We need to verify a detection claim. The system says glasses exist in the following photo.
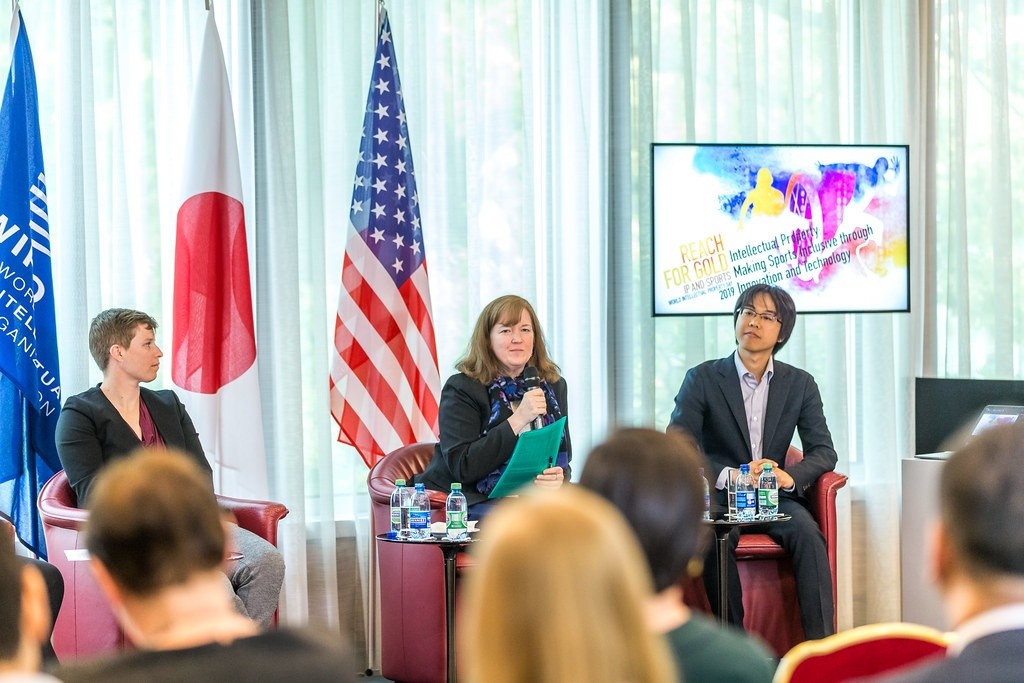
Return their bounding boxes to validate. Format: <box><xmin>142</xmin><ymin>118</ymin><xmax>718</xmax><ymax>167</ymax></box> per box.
<box><xmin>738</xmin><ymin>307</ymin><xmax>782</xmax><ymax>325</ymax></box>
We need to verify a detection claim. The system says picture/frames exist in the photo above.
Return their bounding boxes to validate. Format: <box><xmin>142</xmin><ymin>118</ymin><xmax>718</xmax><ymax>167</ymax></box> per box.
<box><xmin>649</xmin><ymin>141</ymin><xmax>912</xmax><ymax>319</ymax></box>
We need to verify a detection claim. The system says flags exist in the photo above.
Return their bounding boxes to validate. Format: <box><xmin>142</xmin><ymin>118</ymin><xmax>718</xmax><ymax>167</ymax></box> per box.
<box><xmin>161</xmin><ymin>0</ymin><xmax>272</xmax><ymax>504</ymax></box>
<box><xmin>0</xmin><ymin>1</ymin><xmax>64</xmax><ymax>569</ymax></box>
<box><xmin>328</xmin><ymin>1</ymin><xmax>444</xmax><ymax>470</ymax></box>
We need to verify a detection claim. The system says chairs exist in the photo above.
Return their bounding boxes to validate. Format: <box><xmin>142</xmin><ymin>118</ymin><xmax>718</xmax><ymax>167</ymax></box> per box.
<box><xmin>770</xmin><ymin>621</ymin><xmax>951</xmax><ymax>683</ymax></box>
<box><xmin>366</xmin><ymin>442</ymin><xmax>476</xmax><ymax>683</ymax></box>
<box><xmin>36</xmin><ymin>468</ymin><xmax>290</xmax><ymax>670</ymax></box>
<box><xmin>666</xmin><ymin>425</ymin><xmax>848</xmax><ymax>660</ymax></box>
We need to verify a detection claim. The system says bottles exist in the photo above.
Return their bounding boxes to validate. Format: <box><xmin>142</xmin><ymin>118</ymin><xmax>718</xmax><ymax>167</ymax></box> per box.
<box><xmin>699</xmin><ymin>467</ymin><xmax>711</xmax><ymax>520</ymax></box>
<box><xmin>408</xmin><ymin>483</ymin><xmax>430</xmax><ymax>539</ymax></box>
<box><xmin>445</xmin><ymin>483</ymin><xmax>467</xmax><ymax>539</ymax></box>
<box><xmin>757</xmin><ymin>462</ymin><xmax>779</xmax><ymax>515</ymax></box>
<box><xmin>389</xmin><ymin>479</ymin><xmax>411</xmax><ymax>537</ymax></box>
<box><xmin>736</xmin><ymin>465</ymin><xmax>756</xmax><ymax>518</ymax></box>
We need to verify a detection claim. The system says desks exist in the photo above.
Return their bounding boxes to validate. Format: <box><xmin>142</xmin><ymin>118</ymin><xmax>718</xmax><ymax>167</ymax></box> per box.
<box><xmin>696</xmin><ymin>510</ymin><xmax>791</xmax><ymax>634</ymax></box>
<box><xmin>375</xmin><ymin>531</ymin><xmax>479</xmax><ymax>682</ymax></box>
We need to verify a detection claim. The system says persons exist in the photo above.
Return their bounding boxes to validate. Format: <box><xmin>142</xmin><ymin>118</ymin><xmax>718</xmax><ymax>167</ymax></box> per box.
<box><xmin>451</xmin><ymin>426</ymin><xmax>773</xmax><ymax>683</ymax></box>
<box><xmin>0</xmin><ymin>450</ymin><xmax>364</xmax><ymax>683</ymax></box>
<box><xmin>54</xmin><ymin>307</ymin><xmax>284</xmax><ymax>634</ymax></box>
<box><xmin>663</xmin><ymin>283</ymin><xmax>840</xmax><ymax>653</ymax></box>
<box><xmin>879</xmin><ymin>424</ymin><xmax>1024</xmax><ymax>683</ymax></box>
<box><xmin>413</xmin><ymin>296</ymin><xmax>571</xmax><ymax>528</ymax></box>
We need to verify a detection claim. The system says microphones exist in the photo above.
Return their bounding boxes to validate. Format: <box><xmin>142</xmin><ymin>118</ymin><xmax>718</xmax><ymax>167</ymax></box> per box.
<box><xmin>524</xmin><ymin>366</ymin><xmax>543</xmax><ymax>429</ymax></box>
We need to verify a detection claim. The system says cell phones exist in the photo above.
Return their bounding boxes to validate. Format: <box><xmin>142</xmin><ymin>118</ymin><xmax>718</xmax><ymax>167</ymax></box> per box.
<box><xmin>223</xmin><ymin>551</ymin><xmax>244</xmax><ymax>561</ymax></box>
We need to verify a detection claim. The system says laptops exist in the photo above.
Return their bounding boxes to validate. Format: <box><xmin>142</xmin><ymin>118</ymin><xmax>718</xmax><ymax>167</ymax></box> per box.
<box><xmin>915</xmin><ymin>404</ymin><xmax>1024</xmax><ymax>461</ymax></box>
